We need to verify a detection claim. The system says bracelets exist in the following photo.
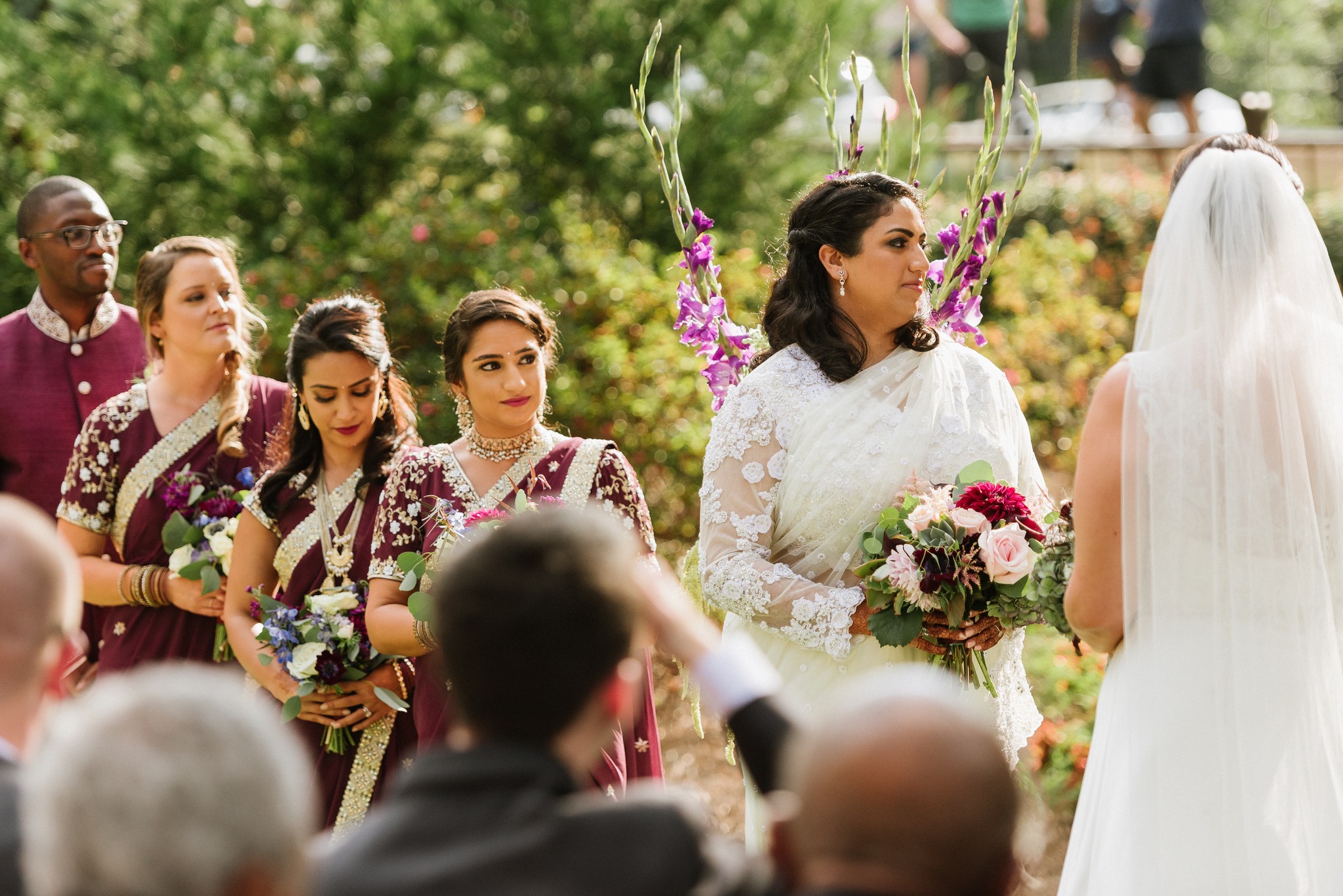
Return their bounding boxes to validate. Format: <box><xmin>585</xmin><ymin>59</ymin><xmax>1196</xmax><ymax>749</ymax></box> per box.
<box><xmin>412</xmin><ymin>618</ymin><xmax>441</xmax><ymax>652</ymax></box>
<box><xmin>387</xmin><ymin>656</ymin><xmax>417</xmax><ymax>699</ymax></box>
<box><xmin>118</xmin><ymin>564</ymin><xmax>169</xmax><ymax>608</ymax></box>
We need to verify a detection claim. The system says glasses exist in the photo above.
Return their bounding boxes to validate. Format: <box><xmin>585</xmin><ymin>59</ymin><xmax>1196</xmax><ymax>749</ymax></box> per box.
<box><xmin>27</xmin><ymin>220</ymin><xmax>127</xmax><ymax>250</ymax></box>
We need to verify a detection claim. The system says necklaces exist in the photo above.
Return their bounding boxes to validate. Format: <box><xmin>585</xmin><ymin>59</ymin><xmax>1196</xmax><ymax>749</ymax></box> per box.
<box><xmin>470</xmin><ymin>423</ymin><xmax>541</xmax><ymax>451</ymax></box>
<box><xmin>315</xmin><ymin>464</ymin><xmax>370</xmax><ymax>595</ymax></box>
<box><xmin>466</xmin><ymin>435</ymin><xmax>542</xmax><ymax>461</ymax></box>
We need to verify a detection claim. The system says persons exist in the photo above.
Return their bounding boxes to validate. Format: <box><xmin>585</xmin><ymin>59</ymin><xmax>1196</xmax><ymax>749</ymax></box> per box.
<box><xmin>769</xmin><ymin>687</ymin><xmax>1023</xmax><ymax>895</ymax></box>
<box><xmin>18</xmin><ymin>662</ymin><xmax>323</xmax><ymax>896</ymax></box>
<box><xmin>1055</xmin><ymin>135</ymin><xmax>1343</xmax><ymax>895</ymax></box>
<box><xmin>220</xmin><ymin>293</ymin><xmax>424</xmax><ymax>838</ymax></box>
<box><xmin>696</xmin><ymin>175</ymin><xmax>1060</xmax><ymax>896</ymax></box>
<box><xmin>0</xmin><ymin>490</ymin><xmax>89</xmax><ymax>896</ymax></box>
<box><xmin>364</xmin><ymin>288</ymin><xmax>666</xmax><ymax>799</ymax></box>
<box><xmin>55</xmin><ymin>232</ymin><xmax>293</xmax><ymax>669</ymax></box>
<box><xmin>310</xmin><ymin>500</ymin><xmax>803</xmax><ymax>896</ymax></box>
<box><xmin>1088</xmin><ymin>0</ymin><xmax>1207</xmax><ymax>139</ymax></box>
<box><xmin>0</xmin><ymin>173</ymin><xmax>160</xmax><ymax>662</ymax></box>
<box><xmin>886</xmin><ymin>0</ymin><xmax>1050</xmax><ymax>137</ymax></box>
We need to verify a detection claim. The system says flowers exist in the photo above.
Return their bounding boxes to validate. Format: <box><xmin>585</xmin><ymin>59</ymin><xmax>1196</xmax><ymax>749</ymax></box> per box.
<box><xmin>159</xmin><ymin>459</ymin><xmax>266</xmax><ymax>671</ymax></box>
<box><xmin>243</xmin><ymin>566</ymin><xmax>413</xmax><ymax>760</ymax></box>
<box><xmin>625</xmin><ymin>0</ymin><xmax>1046</xmax><ymax>408</ymax></box>
<box><xmin>860</xmin><ymin>462</ymin><xmax>1047</xmax><ymax>711</ymax></box>
<box><xmin>399</xmin><ymin>460</ymin><xmax>589</xmax><ymax>630</ymax></box>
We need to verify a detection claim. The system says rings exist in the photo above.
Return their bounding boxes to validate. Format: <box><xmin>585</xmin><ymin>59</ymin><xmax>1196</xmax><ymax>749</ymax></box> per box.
<box><xmin>363</xmin><ymin>706</ymin><xmax>372</xmax><ymax>717</ymax></box>
<box><xmin>920</xmin><ymin>627</ymin><xmax>930</xmax><ymax>638</ymax></box>
<box><xmin>993</xmin><ymin>624</ymin><xmax>1003</xmax><ymax>636</ymax></box>
<box><xmin>209</xmin><ymin>599</ymin><xmax>214</xmax><ymax>608</ymax></box>
